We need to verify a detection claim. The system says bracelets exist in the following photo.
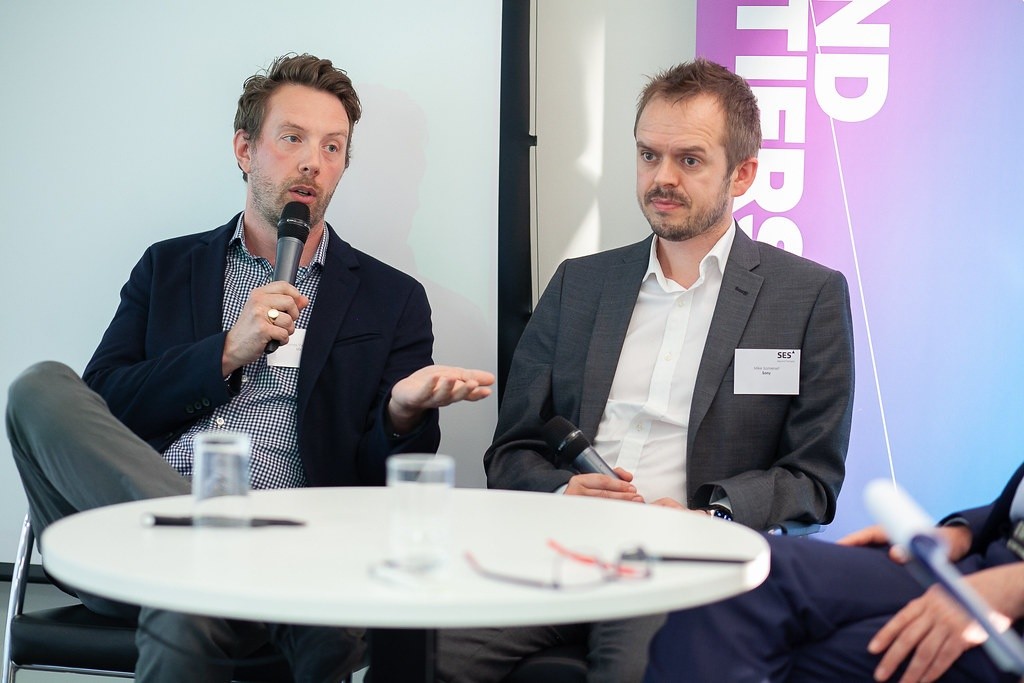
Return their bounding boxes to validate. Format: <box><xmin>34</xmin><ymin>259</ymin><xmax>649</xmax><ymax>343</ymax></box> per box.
<box><xmin>224</xmin><ymin>373</ymin><xmax>232</xmax><ymax>382</ymax></box>
<box><xmin>391</xmin><ymin>432</ymin><xmax>402</xmax><ymax>438</ymax></box>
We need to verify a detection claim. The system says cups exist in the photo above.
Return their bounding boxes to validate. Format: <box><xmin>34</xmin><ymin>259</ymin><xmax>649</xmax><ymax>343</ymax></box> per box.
<box><xmin>386</xmin><ymin>455</ymin><xmax>452</xmax><ymax>557</ymax></box>
<box><xmin>193</xmin><ymin>430</ymin><xmax>255</xmax><ymax>550</ymax></box>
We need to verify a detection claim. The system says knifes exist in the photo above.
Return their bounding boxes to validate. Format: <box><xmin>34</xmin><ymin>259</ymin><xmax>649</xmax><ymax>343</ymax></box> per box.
<box><xmin>146</xmin><ymin>513</ymin><xmax>302</xmax><ymax>528</ymax></box>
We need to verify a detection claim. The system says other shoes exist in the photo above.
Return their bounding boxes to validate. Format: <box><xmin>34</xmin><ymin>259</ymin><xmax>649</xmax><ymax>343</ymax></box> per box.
<box><xmin>276</xmin><ymin>627</ymin><xmax>368</xmax><ymax>683</ymax></box>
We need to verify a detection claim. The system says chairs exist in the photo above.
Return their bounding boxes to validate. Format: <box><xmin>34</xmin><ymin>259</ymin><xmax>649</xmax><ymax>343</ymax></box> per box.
<box><xmin>1</xmin><ymin>513</ymin><xmax>141</xmax><ymax>683</ymax></box>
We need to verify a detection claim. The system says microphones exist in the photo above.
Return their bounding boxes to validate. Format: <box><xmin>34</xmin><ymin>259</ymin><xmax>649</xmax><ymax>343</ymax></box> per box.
<box><xmin>539</xmin><ymin>415</ymin><xmax>623</xmax><ymax>481</ymax></box>
<box><xmin>264</xmin><ymin>200</ymin><xmax>310</xmax><ymax>355</ymax></box>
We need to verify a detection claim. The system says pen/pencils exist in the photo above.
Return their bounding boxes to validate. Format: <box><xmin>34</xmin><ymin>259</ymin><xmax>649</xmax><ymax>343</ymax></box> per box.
<box><xmin>625</xmin><ymin>549</ymin><xmax>755</xmax><ymax>565</ymax></box>
<box><xmin>144</xmin><ymin>516</ymin><xmax>307</xmax><ymax>528</ymax></box>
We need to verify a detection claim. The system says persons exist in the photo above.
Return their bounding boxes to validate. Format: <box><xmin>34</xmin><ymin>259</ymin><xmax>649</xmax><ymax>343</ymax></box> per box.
<box><xmin>4</xmin><ymin>50</ymin><xmax>494</xmax><ymax>683</ymax></box>
<box><xmin>642</xmin><ymin>460</ymin><xmax>1024</xmax><ymax>683</ymax></box>
<box><xmin>431</xmin><ymin>58</ymin><xmax>855</xmax><ymax>683</ymax></box>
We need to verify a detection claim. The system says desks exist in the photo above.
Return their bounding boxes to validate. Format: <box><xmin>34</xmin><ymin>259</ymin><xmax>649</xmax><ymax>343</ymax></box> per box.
<box><xmin>42</xmin><ymin>487</ymin><xmax>772</xmax><ymax>683</ymax></box>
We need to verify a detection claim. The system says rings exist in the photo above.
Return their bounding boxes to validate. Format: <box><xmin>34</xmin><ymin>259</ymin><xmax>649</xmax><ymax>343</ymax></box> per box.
<box><xmin>267</xmin><ymin>309</ymin><xmax>279</xmax><ymax>320</ymax></box>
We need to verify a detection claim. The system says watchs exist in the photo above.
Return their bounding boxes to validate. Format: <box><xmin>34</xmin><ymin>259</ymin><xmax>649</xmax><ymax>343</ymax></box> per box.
<box><xmin>698</xmin><ymin>507</ymin><xmax>733</xmax><ymax>522</ymax></box>
